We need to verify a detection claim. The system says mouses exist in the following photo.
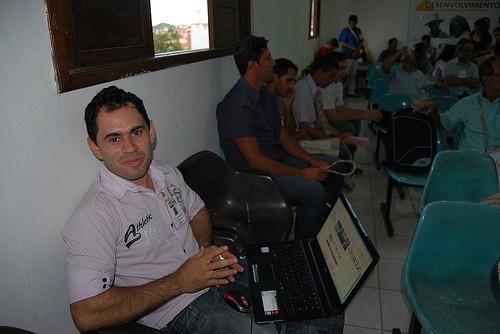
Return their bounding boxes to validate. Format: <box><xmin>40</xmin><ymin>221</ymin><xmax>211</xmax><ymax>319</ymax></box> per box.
<box><xmin>224</xmin><ymin>290</ymin><xmax>252</xmax><ymax>313</ymax></box>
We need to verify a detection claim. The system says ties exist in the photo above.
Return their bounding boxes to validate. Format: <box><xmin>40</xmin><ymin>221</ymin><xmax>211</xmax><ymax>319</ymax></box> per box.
<box><xmin>314</xmin><ymin>90</ymin><xmax>332</xmax><ymax>135</ymax></box>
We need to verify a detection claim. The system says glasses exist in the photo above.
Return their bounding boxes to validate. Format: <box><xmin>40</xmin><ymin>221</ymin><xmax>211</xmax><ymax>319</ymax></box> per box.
<box><xmin>338</xmin><ymin>65</ymin><xmax>347</xmax><ymax>71</ymax></box>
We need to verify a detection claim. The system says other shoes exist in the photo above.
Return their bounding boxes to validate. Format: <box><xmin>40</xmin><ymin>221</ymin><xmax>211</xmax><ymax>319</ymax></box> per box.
<box><xmin>346</xmin><ymin>94</ymin><xmax>359</xmax><ymax>99</ymax></box>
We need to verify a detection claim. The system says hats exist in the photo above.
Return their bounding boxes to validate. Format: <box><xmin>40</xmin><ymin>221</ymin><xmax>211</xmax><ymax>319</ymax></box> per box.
<box><xmin>423</xmin><ymin>13</ymin><xmax>444</xmax><ymax>25</ymax></box>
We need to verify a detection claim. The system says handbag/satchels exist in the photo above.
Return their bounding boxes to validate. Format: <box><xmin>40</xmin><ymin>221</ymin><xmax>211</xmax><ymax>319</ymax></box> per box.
<box><xmin>387</xmin><ymin>108</ymin><xmax>437</xmax><ymax>174</ymax></box>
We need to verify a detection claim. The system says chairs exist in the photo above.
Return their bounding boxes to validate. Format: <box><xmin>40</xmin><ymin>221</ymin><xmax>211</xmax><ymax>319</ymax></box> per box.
<box><xmin>89</xmin><ymin>62</ymin><xmax>499</xmax><ymax>334</ymax></box>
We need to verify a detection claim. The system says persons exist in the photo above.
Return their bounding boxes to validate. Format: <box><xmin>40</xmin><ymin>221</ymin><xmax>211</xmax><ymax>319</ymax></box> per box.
<box><xmin>267</xmin><ymin>15</ymin><xmax>500</xmax><ymax>191</ymax></box>
<box><xmin>216</xmin><ymin>36</ymin><xmax>338</xmax><ymax>240</ymax></box>
<box><xmin>62</xmin><ymin>87</ymin><xmax>340</xmax><ymax>334</ymax></box>
<box><xmin>413</xmin><ymin>56</ymin><xmax>500</xmax><ymax>151</ymax></box>
<box><xmin>422</xmin><ymin>13</ymin><xmax>490</xmax><ymax>38</ymax></box>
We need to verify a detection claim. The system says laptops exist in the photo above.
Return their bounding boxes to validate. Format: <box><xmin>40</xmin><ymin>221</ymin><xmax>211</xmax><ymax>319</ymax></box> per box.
<box><xmin>244</xmin><ymin>191</ymin><xmax>380</xmax><ymax>325</ymax></box>
